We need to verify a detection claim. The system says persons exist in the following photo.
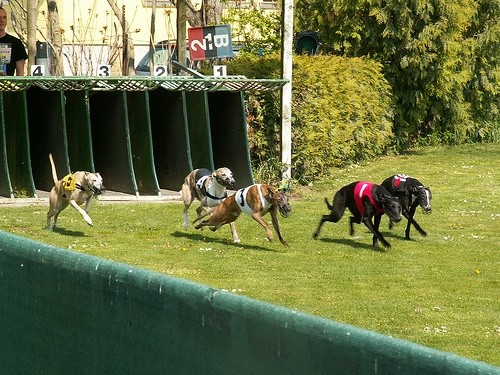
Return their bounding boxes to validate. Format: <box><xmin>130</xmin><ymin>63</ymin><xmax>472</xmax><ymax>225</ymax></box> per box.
<box><xmin>0</xmin><ymin>7</ymin><xmax>29</xmax><ymax>76</ymax></box>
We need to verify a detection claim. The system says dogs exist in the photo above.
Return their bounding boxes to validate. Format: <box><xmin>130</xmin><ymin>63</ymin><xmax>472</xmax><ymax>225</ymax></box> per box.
<box><xmin>192</xmin><ymin>183</ymin><xmax>292</xmax><ymax>247</ymax></box>
<box><xmin>312</xmin><ymin>181</ymin><xmax>403</xmax><ymax>252</ymax></box>
<box><xmin>181</xmin><ymin>167</ymin><xmax>241</xmax><ymax>243</ymax></box>
<box><xmin>46</xmin><ymin>153</ymin><xmax>106</xmax><ymax>231</ymax></box>
<box><xmin>381</xmin><ymin>173</ymin><xmax>432</xmax><ymax>241</ymax></box>
